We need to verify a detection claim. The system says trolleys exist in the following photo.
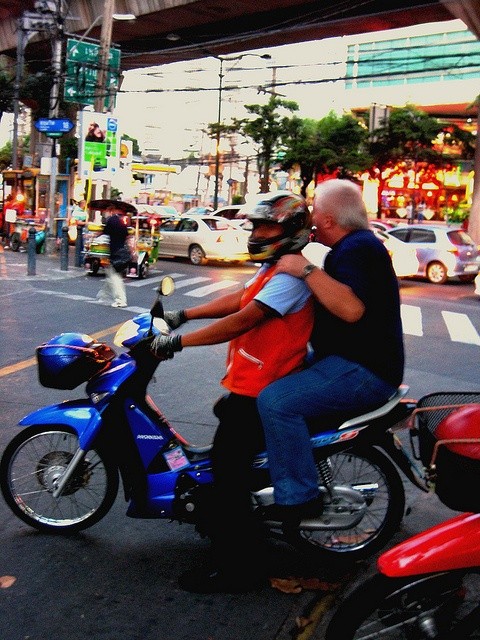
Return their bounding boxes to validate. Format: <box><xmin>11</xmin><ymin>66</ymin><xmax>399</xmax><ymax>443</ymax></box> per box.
<box><xmin>7</xmin><ymin>218</ymin><xmax>50</xmax><ymax>252</ymax></box>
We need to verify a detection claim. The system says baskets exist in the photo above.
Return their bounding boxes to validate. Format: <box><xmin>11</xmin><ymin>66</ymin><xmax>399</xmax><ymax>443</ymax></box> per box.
<box><xmin>37</xmin><ymin>344</ymin><xmax>103</xmax><ymax>388</ymax></box>
<box><xmin>416</xmin><ymin>392</ymin><xmax>479</xmax><ymax>513</ymax></box>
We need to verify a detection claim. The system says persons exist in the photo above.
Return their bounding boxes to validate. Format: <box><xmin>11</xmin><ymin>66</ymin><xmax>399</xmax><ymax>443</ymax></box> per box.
<box><xmin>255</xmin><ymin>177</ymin><xmax>405</xmax><ymax>524</ymax></box>
<box><xmin>96</xmin><ymin>205</ymin><xmax>128</xmax><ymax>308</ymax></box>
<box><xmin>417</xmin><ymin>199</ymin><xmax>426</xmax><ymax>223</ymax></box>
<box><xmin>71</xmin><ymin>200</ymin><xmax>89</xmax><ymax>268</ymax></box>
<box><xmin>149</xmin><ymin>189</ymin><xmax>313</xmax><ymax>577</ymax></box>
<box><xmin>405</xmin><ymin>201</ymin><xmax>414</xmax><ymax>225</ymax></box>
<box><xmin>85</xmin><ymin>122</ymin><xmax>107</xmax><ymax>142</ymax></box>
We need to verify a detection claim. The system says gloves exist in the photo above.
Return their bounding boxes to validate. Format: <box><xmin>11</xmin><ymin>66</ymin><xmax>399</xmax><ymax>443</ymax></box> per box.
<box><xmin>164</xmin><ymin>310</ymin><xmax>184</xmax><ymax>327</ymax></box>
<box><xmin>146</xmin><ymin>335</ymin><xmax>183</xmax><ymax>360</ymax></box>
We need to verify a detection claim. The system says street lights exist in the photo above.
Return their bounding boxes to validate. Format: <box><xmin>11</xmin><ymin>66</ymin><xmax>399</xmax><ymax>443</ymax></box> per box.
<box><xmin>208</xmin><ymin>51</ymin><xmax>272</xmax><ymax>210</ymax></box>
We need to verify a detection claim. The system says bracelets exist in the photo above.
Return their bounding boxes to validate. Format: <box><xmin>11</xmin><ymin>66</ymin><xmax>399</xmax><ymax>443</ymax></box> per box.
<box><xmin>301</xmin><ymin>263</ymin><xmax>316</xmax><ymax>279</ymax></box>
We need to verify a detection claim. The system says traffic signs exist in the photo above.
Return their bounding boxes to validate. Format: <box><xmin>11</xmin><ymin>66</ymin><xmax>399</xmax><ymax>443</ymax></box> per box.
<box><xmin>35</xmin><ymin>117</ymin><xmax>75</xmax><ymax>133</ymax></box>
<box><xmin>63</xmin><ymin>60</ymin><xmax>120</xmax><ymax>89</ymax></box>
<box><xmin>64</xmin><ymin>39</ymin><xmax>123</xmax><ymax>70</ymax></box>
<box><xmin>62</xmin><ymin>78</ymin><xmax>117</xmax><ymax>109</ymax></box>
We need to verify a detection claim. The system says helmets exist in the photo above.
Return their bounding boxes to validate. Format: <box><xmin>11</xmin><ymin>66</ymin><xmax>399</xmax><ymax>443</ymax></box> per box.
<box><xmin>248</xmin><ymin>195</ymin><xmax>310</xmax><ymax>261</ymax></box>
<box><xmin>433</xmin><ymin>404</ymin><xmax>479</xmax><ymax>459</ymax></box>
<box><xmin>42</xmin><ymin>332</ymin><xmax>95</xmax><ymax>372</ymax></box>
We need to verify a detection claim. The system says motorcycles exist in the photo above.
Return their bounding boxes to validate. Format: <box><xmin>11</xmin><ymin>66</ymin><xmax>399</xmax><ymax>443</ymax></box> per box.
<box><xmin>311</xmin><ymin>391</ymin><xmax>479</xmax><ymax>640</ymax></box>
<box><xmin>0</xmin><ymin>276</ymin><xmax>431</xmax><ymax>564</ymax></box>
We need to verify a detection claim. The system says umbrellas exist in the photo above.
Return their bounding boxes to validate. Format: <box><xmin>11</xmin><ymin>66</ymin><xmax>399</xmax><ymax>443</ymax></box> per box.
<box><xmin>87</xmin><ymin>198</ymin><xmax>138</xmax><ymax>217</ymax></box>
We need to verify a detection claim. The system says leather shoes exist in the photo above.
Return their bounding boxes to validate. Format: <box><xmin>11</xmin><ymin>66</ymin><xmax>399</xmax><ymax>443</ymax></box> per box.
<box><xmin>261</xmin><ymin>498</ymin><xmax>323</xmax><ymax>518</ymax></box>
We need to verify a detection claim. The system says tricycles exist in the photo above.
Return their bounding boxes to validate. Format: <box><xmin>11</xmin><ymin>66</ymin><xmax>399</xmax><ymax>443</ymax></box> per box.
<box><xmin>85</xmin><ymin>212</ymin><xmax>161</xmax><ymax>279</ymax></box>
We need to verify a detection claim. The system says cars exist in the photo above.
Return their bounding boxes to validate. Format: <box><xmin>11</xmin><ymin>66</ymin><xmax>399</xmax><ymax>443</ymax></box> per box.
<box><xmin>387</xmin><ymin>224</ymin><xmax>480</xmax><ymax>284</ymax></box>
<box><xmin>179</xmin><ymin>206</ymin><xmax>215</xmax><ymax>217</ymax></box>
<box><xmin>368</xmin><ymin>220</ymin><xmax>396</xmax><ymax>232</ymax></box>
<box><xmin>151</xmin><ymin>215</ymin><xmax>251</xmax><ymax>266</ymax></box>
<box><xmin>291</xmin><ymin>228</ymin><xmax>420</xmax><ymax>280</ymax></box>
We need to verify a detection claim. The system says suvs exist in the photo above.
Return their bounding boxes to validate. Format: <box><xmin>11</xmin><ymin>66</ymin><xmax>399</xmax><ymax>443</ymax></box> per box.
<box><xmin>209</xmin><ymin>204</ymin><xmax>271</xmax><ymax>232</ymax></box>
